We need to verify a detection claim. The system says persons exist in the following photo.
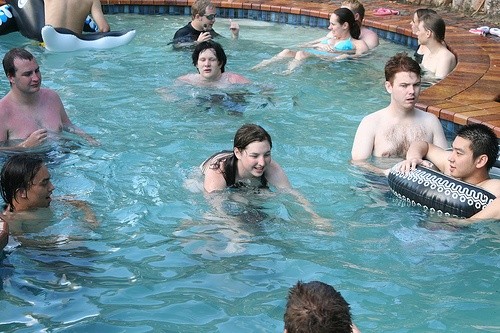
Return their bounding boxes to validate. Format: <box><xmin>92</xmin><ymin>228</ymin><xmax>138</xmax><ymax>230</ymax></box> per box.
<box><xmin>281</xmin><ymin>8</ymin><xmax>370</xmax><ymax>58</ymax></box>
<box><xmin>0</xmin><ymin>152</ymin><xmax>55</xmax><ymax>210</ymax></box>
<box><xmin>397</xmin><ymin>123</ymin><xmax>500</xmax><ymax>218</ymax></box>
<box><xmin>173</xmin><ymin>0</ymin><xmax>239</xmax><ymax>42</ymax></box>
<box><xmin>39</xmin><ymin>0</ymin><xmax>111</xmax><ymax>34</ymax></box>
<box><xmin>0</xmin><ymin>48</ymin><xmax>70</xmax><ymax>147</ymax></box>
<box><xmin>342</xmin><ymin>0</ymin><xmax>380</xmax><ymax>49</ymax></box>
<box><xmin>410</xmin><ymin>10</ymin><xmax>456</xmax><ymax>79</ymax></box>
<box><xmin>177</xmin><ymin>41</ymin><xmax>239</xmax><ymax>84</ymax></box>
<box><xmin>350</xmin><ymin>53</ymin><xmax>448</xmax><ymax>160</ymax></box>
<box><xmin>283</xmin><ymin>280</ymin><xmax>353</xmax><ymax>333</ymax></box>
<box><xmin>201</xmin><ymin>123</ymin><xmax>292</xmax><ymax>192</ymax></box>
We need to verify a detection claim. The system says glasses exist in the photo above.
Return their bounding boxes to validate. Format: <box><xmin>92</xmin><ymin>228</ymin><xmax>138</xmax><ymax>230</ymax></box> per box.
<box><xmin>204</xmin><ymin>13</ymin><xmax>216</xmax><ymax>20</ymax></box>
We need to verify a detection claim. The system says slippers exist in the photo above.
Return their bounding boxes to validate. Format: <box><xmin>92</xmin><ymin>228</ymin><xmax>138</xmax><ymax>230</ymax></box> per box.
<box><xmin>373</xmin><ymin>8</ymin><xmax>399</xmax><ymax>15</ymax></box>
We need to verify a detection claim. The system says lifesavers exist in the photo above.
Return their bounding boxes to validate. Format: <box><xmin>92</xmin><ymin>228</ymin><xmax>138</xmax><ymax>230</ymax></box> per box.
<box><xmin>0</xmin><ymin>0</ymin><xmax>139</xmax><ymax>54</ymax></box>
<box><xmin>387</xmin><ymin>157</ymin><xmax>496</xmax><ymax>222</ymax></box>
